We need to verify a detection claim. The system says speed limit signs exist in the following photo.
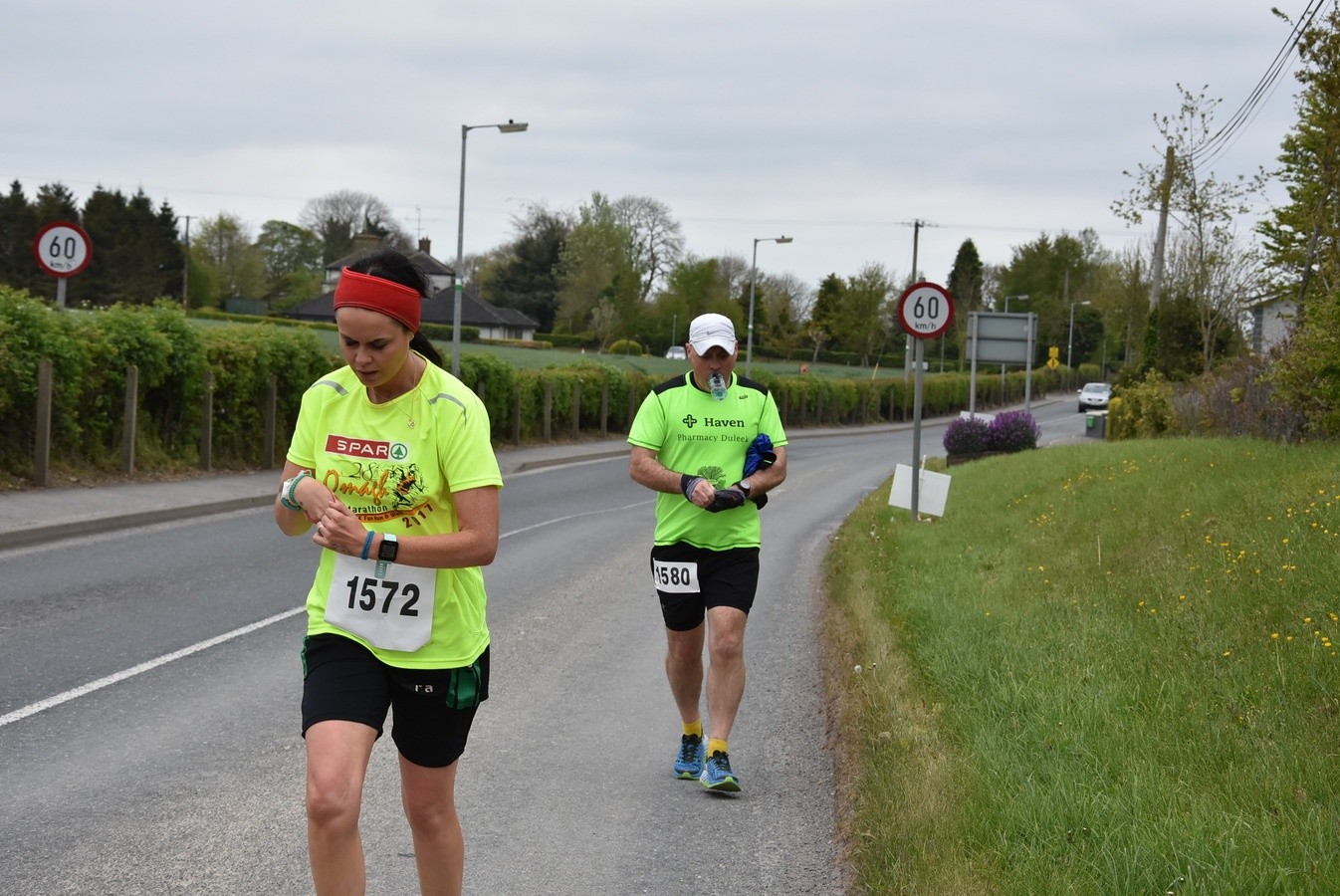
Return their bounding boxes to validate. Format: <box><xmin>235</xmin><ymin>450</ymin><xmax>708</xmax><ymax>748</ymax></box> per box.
<box><xmin>34</xmin><ymin>222</ymin><xmax>92</xmax><ymax>277</ymax></box>
<box><xmin>897</xmin><ymin>282</ymin><xmax>953</xmax><ymax>338</ymax></box>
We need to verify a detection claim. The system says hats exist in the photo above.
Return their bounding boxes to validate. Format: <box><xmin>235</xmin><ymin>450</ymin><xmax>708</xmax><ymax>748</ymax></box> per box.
<box><xmin>689</xmin><ymin>313</ymin><xmax>736</xmax><ymax>357</ymax></box>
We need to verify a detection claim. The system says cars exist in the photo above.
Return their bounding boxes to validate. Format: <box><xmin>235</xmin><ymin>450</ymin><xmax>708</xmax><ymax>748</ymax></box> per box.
<box><xmin>1077</xmin><ymin>382</ymin><xmax>1111</xmax><ymax>414</ymax></box>
<box><xmin>665</xmin><ymin>346</ymin><xmax>687</xmax><ymax>360</ymax></box>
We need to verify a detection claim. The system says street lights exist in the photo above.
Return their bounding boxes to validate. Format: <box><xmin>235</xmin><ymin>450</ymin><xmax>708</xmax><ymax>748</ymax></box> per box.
<box><xmin>451</xmin><ymin>120</ymin><xmax>530</xmax><ymax>380</ymax></box>
<box><xmin>999</xmin><ymin>295</ymin><xmax>1029</xmax><ymax>405</ymax></box>
<box><xmin>745</xmin><ymin>236</ymin><xmax>793</xmax><ymax>380</ymax></box>
<box><xmin>1064</xmin><ymin>301</ymin><xmax>1091</xmax><ymax>392</ymax></box>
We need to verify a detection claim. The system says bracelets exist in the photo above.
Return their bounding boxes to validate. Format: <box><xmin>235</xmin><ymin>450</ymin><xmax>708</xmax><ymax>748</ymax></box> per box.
<box><xmin>279</xmin><ymin>476</ymin><xmax>301</xmax><ymax>510</ymax></box>
<box><xmin>361</xmin><ymin>530</ymin><xmax>374</xmax><ymax>560</ymax></box>
<box><xmin>288</xmin><ymin>473</ymin><xmax>312</xmax><ymax>505</ymax></box>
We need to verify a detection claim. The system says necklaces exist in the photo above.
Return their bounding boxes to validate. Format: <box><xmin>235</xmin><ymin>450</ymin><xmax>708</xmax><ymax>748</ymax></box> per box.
<box><xmin>371</xmin><ymin>349</ymin><xmax>416</xmax><ymax>429</ymax></box>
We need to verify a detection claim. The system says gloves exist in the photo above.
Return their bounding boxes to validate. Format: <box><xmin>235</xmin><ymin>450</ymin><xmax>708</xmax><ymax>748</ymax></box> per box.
<box><xmin>705</xmin><ymin>489</ymin><xmax>745</xmax><ymax>513</ymax></box>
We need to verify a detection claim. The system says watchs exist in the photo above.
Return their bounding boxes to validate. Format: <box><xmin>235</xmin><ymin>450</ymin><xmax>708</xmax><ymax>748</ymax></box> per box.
<box><xmin>375</xmin><ymin>534</ymin><xmax>399</xmax><ymax>579</ymax></box>
<box><xmin>731</xmin><ymin>480</ymin><xmax>751</xmax><ymax>498</ymax></box>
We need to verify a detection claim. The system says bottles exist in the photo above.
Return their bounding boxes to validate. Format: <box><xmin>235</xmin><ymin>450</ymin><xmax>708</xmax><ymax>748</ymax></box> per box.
<box><xmin>708</xmin><ymin>374</ymin><xmax>727</xmax><ymax>400</ymax></box>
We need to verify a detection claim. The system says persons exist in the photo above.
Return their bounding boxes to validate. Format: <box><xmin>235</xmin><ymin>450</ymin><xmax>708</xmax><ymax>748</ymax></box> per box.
<box><xmin>625</xmin><ymin>313</ymin><xmax>788</xmax><ymax>792</ymax></box>
<box><xmin>274</xmin><ymin>246</ymin><xmax>506</xmax><ymax>896</ymax></box>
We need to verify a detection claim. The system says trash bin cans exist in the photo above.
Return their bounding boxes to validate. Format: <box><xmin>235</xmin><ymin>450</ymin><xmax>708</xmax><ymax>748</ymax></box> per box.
<box><xmin>1085</xmin><ymin>410</ymin><xmax>1108</xmax><ymax>440</ymax></box>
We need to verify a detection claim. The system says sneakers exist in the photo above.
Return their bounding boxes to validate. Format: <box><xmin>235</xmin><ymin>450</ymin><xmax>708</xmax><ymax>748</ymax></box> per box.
<box><xmin>672</xmin><ymin>724</ymin><xmax>710</xmax><ymax>780</ymax></box>
<box><xmin>699</xmin><ymin>749</ymin><xmax>742</xmax><ymax>791</ymax></box>
